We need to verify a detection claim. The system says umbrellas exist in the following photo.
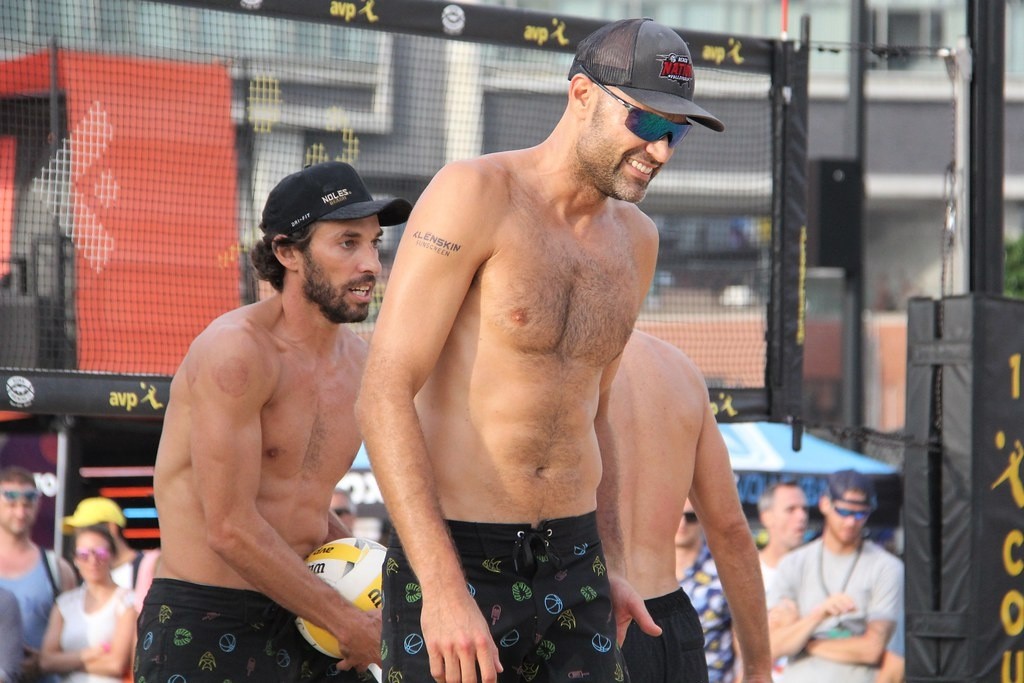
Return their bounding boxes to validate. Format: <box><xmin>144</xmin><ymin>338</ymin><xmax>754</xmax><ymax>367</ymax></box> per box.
<box><xmin>717</xmin><ymin>422</ymin><xmax>897</xmax><ymax>509</ymax></box>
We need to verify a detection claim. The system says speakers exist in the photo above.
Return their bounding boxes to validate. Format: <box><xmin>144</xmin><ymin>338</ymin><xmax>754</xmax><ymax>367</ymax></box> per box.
<box><xmin>805</xmin><ymin>158</ymin><xmax>861</xmax><ymax>268</ymax></box>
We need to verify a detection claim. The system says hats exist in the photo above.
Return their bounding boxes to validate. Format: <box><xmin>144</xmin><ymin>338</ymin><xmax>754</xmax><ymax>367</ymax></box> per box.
<box><xmin>829</xmin><ymin>470</ymin><xmax>870</xmax><ymax>504</ymax></box>
<box><xmin>566</xmin><ymin>18</ymin><xmax>726</xmax><ymax>133</ymax></box>
<box><xmin>64</xmin><ymin>496</ymin><xmax>127</xmax><ymax>536</ymax></box>
<box><xmin>263</xmin><ymin>163</ymin><xmax>413</xmax><ymax>237</ymax></box>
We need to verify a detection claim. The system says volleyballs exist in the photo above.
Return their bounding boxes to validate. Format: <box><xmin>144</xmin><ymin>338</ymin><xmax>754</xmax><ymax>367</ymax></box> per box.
<box><xmin>294</xmin><ymin>537</ymin><xmax>388</xmax><ymax>660</ymax></box>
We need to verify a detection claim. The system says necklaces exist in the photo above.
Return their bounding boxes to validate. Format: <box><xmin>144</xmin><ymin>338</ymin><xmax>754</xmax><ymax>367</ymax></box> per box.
<box><xmin>818</xmin><ymin>538</ymin><xmax>865</xmax><ymax>597</ymax></box>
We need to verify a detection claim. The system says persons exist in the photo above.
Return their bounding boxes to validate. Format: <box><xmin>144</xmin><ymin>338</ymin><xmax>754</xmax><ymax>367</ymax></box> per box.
<box><xmin>0</xmin><ymin>464</ymin><xmax>161</xmax><ymax>683</ymax></box>
<box><xmin>755</xmin><ymin>476</ymin><xmax>808</xmax><ymax>592</ymax></box>
<box><xmin>355</xmin><ymin>18</ymin><xmax>725</xmax><ymax>683</ymax></box>
<box><xmin>609</xmin><ymin>330</ymin><xmax>772</xmax><ymax>683</ymax></box>
<box><xmin>134</xmin><ymin>162</ymin><xmax>413</xmax><ymax>683</ymax></box>
<box><xmin>673</xmin><ymin>497</ymin><xmax>736</xmax><ymax>683</ymax></box>
<box><xmin>322</xmin><ymin>487</ymin><xmax>357</xmax><ymax>544</ymax></box>
<box><xmin>767</xmin><ymin>466</ymin><xmax>904</xmax><ymax>683</ymax></box>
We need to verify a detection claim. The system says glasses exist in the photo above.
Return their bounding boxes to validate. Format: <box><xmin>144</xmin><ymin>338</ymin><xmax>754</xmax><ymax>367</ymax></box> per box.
<box><xmin>2</xmin><ymin>490</ymin><xmax>43</xmax><ymax>501</ymax></box>
<box><xmin>72</xmin><ymin>548</ymin><xmax>112</xmax><ymax>561</ymax></box>
<box><xmin>682</xmin><ymin>513</ymin><xmax>696</xmax><ymax>522</ymax></box>
<box><xmin>577</xmin><ymin>65</ymin><xmax>694</xmax><ymax>150</ymax></box>
<box><xmin>829</xmin><ymin>497</ymin><xmax>870</xmax><ymax>521</ymax></box>
